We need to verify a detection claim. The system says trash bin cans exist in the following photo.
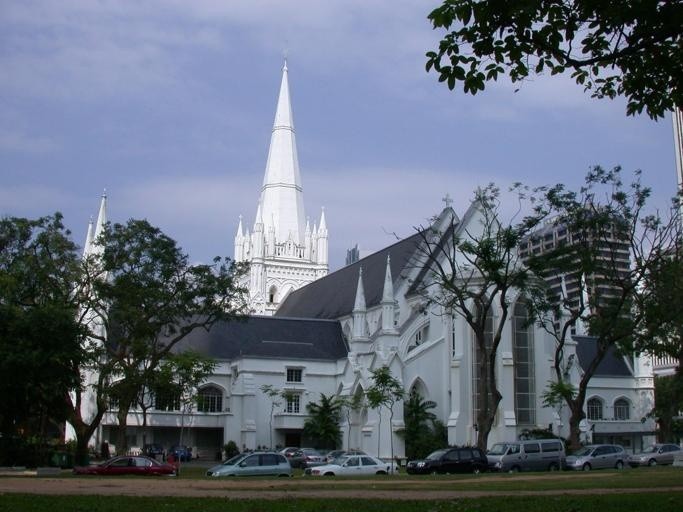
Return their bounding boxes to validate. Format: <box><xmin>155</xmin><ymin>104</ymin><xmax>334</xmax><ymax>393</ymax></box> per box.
<box><xmin>52</xmin><ymin>450</ymin><xmax>76</xmax><ymax>469</ymax></box>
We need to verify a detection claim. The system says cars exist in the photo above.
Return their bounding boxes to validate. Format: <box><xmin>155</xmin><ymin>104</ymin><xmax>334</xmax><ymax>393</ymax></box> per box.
<box><xmin>279</xmin><ymin>446</ymin><xmax>400</xmax><ymax>477</ymax></box>
<box><xmin>566</xmin><ymin>443</ymin><xmax>628</xmax><ymax>472</ymax></box>
<box><xmin>204</xmin><ymin>450</ymin><xmax>293</xmax><ymax>478</ymax></box>
<box><xmin>167</xmin><ymin>445</ymin><xmax>193</xmax><ymax>462</ymax></box>
<box><xmin>141</xmin><ymin>443</ymin><xmax>165</xmax><ymax>460</ymax></box>
<box><xmin>627</xmin><ymin>442</ymin><xmax>681</xmax><ymax>467</ymax></box>
<box><xmin>71</xmin><ymin>449</ymin><xmax>178</xmax><ymax>476</ymax></box>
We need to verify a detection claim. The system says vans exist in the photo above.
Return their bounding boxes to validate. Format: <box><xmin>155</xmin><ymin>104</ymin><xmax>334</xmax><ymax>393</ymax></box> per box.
<box><xmin>486</xmin><ymin>438</ymin><xmax>566</xmax><ymax>474</ymax></box>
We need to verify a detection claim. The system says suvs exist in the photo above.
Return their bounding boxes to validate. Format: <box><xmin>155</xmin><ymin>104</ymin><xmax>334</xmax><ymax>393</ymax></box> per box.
<box><xmin>406</xmin><ymin>446</ymin><xmax>489</xmax><ymax>475</ymax></box>
